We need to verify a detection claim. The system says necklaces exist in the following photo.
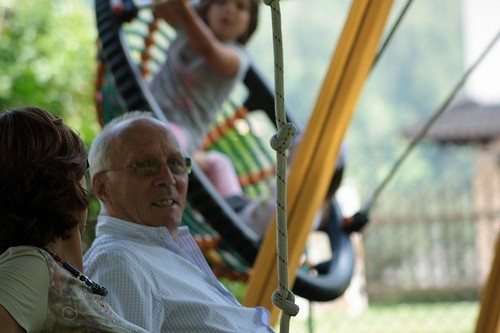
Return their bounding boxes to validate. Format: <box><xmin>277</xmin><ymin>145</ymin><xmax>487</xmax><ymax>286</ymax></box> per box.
<box><xmin>46</xmin><ymin>248</ymin><xmax>108</xmax><ymax>297</ymax></box>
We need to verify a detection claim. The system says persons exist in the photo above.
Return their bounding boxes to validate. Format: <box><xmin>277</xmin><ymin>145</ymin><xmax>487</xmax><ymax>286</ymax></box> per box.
<box><xmin>0</xmin><ymin>106</ymin><xmax>149</xmax><ymax>333</ymax></box>
<box><xmin>83</xmin><ymin>111</ymin><xmax>276</xmax><ymax>333</ymax></box>
<box><xmin>149</xmin><ymin>0</ymin><xmax>258</xmax><ymax>212</ymax></box>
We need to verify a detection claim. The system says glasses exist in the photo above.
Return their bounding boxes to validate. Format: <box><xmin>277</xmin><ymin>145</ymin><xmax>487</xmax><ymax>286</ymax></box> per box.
<box><xmin>98</xmin><ymin>152</ymin><xmax>192</xmax><ymax>179</ymax></box>
